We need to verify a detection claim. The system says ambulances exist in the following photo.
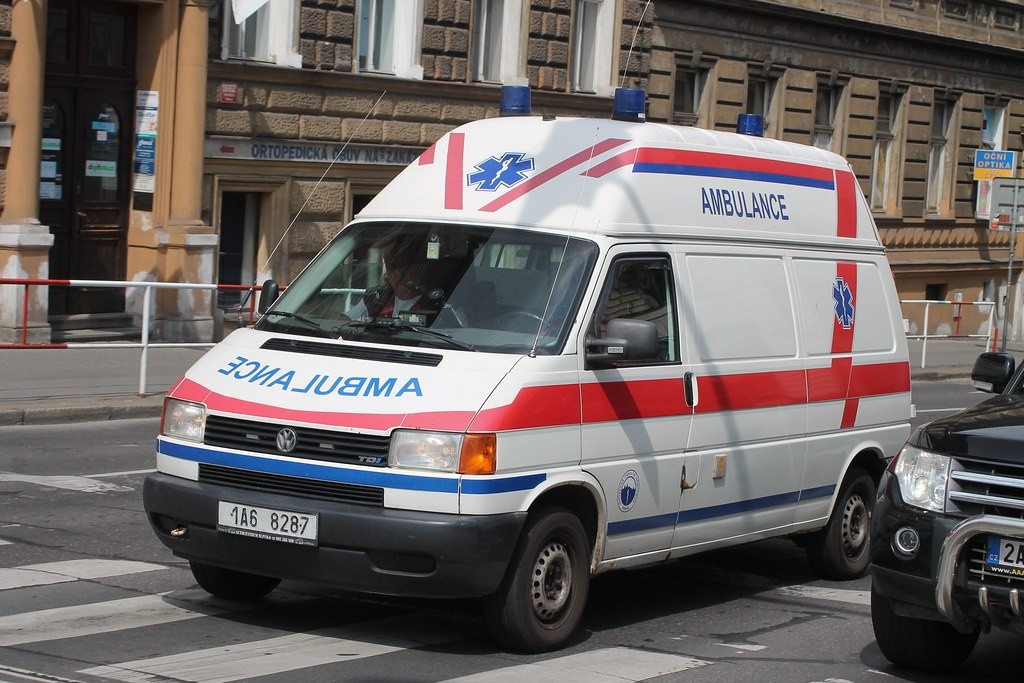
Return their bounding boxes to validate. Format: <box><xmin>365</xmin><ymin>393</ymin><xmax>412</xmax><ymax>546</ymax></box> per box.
<box><xmin>141</xmin><ymin>86</ymin><xmax>915</xmax><ymax>652</ymax></box>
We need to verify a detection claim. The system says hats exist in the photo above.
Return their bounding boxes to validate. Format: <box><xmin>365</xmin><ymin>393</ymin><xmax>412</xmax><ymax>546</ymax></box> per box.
<box><xmin>383</xmin><ymin>231</ymin><xmax>428</xmax><ymax>266</ymax></box>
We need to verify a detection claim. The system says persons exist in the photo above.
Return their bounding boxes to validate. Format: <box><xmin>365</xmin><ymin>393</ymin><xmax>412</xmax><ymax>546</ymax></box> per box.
<box><xmin>300</xmin><ymin>246</ymin><xmax>469</xmax><ymax>330</ymax></box>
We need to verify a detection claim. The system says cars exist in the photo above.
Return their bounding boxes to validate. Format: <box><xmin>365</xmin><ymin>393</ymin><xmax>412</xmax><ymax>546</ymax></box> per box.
<box><xmin>868</xmin><ymin>351</ymin><xmax>1024</xmax><ymax>672</ymax></box>
